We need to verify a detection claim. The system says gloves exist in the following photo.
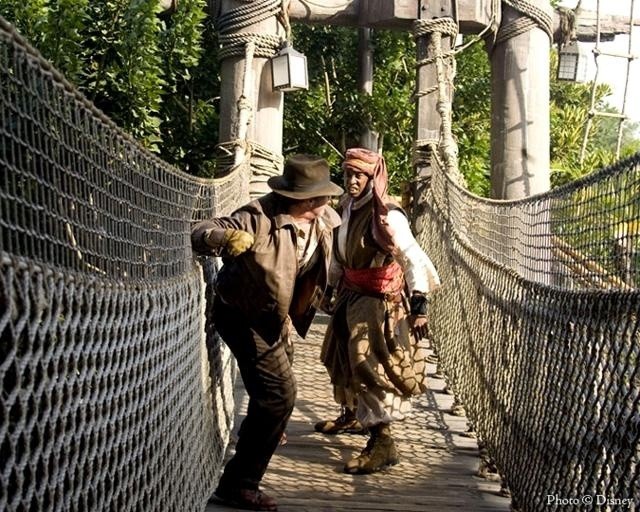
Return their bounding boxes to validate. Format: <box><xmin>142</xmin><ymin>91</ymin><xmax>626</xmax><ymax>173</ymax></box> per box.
<box><xmin>219</xmin><ymin>227</ymin><xmax>254</xmax><ymax>258</ymax></box>
<box><xmin>331</xmin><ymin>196</ymin><xmax>342</xmax><ymax>209</ymax></box>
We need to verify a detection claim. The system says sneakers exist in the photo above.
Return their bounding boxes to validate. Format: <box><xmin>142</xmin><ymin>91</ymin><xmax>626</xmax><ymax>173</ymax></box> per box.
<box><xmin>214</xmin><ymin>474</ymin><xmax>278</xmax><ymax>512</ymax></box>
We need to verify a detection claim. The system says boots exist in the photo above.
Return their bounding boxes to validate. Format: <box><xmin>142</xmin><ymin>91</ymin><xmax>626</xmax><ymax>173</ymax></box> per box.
<box><xmin>343</xmin><ymin>423</ymin><xmax>400</xmax><ymax>474</ymax></box>
<box><xmin>314</xmin><ymin>405</ymin><xmax>368</xmax><ymax>435</ymax></box>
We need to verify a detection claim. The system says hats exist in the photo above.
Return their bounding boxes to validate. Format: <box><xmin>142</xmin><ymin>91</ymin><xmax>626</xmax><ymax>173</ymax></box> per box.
<box><xmin>268</xmin><ymin>154</ymin><xmax>345</xmax><ymax>201</ymax></box>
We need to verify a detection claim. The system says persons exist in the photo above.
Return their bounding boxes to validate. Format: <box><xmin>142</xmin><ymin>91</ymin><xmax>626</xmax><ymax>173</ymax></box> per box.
<box><xmin>189</xmin><ymin>153</ymin><xmax>350</xmax><ymax>511</ymax></box>
<box><xmin>311</xmin><ymin>145</ymin><xmax>445</xmax><ymax>477</ymax></box>
